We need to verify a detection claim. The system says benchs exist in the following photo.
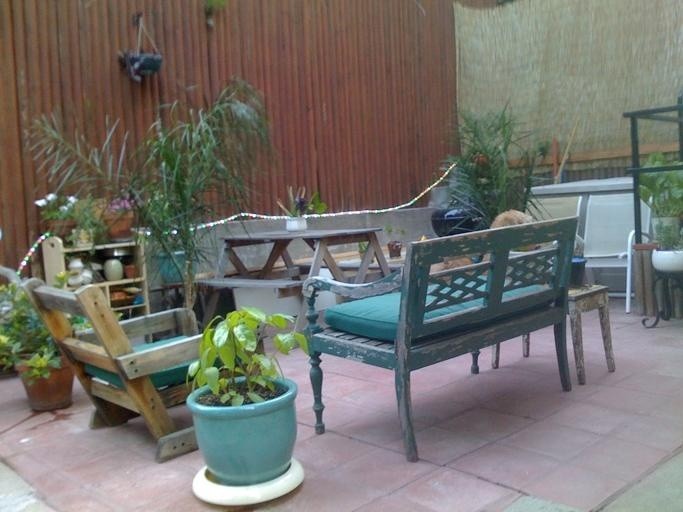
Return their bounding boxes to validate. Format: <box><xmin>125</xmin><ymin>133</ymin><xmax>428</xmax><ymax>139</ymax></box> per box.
<box><xmin>301</xmin><ymin>215</ymin><xmax>578</xmax><ymax>462</ymax></box>
<box><xmin>194</xmin><ymin>261</ymin><xmax>404</xmax><ymax>350</ymax></box>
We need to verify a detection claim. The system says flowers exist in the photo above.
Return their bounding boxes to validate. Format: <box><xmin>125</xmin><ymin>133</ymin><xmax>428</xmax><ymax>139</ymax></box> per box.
<box><xmin>33</xmin><ymin>193</ymin><xmax>79</xmax><ymax>219</ymax></box>
<box><xmin>107</xmin><ymin>190</ymin><xmax>136</xmax><ymax>212</ymax></box>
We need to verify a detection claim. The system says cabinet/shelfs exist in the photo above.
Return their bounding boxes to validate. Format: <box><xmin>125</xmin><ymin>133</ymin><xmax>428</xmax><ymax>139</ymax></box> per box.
<box><xmin>41</xmin><ymin>228</ymin><xmax>150</xmax><ymax>322</ymax></box>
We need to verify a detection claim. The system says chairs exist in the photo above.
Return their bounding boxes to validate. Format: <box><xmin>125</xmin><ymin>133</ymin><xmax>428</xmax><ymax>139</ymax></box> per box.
<box><xmin>20</xmin><ymin>277</ymin><xmax>269</xmax><ymax>464</ymax></box>
<box><xmin>508</xmin><ymin>193</ymin><xmax>652</xmax><ymax>314</ymax></box>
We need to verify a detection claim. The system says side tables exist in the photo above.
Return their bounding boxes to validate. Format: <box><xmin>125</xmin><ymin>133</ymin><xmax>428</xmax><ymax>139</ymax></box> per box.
<box><xmin>492</xmin><ymin>284</ymin><xmax>615</xmax><ymax>385</ymax></box>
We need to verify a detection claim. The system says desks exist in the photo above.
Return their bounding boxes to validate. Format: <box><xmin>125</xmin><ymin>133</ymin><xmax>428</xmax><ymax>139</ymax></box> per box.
<box><xmin>202</xmin><ymin>228</ymin><xmax>400</xmax><ymax>350</ymax></box>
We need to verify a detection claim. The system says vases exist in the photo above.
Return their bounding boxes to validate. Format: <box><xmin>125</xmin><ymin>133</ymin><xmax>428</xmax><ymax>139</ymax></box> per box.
<box><xmin>551</xmin><ymin>257</ymin><xmax>587</xmax><ymax>289</ymax></box>
<box><xmin>102</xmin><ymin>212</ymin><xmax>135</xmax><ymax>237</ymax></box>
<box><xmin>45</xmin><ymin>219</ymin><xmax>77</xmax><ymax>243</ymax></box>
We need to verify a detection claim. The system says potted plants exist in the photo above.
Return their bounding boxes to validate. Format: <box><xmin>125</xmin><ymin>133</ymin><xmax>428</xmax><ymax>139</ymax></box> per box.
<box><xmin>274</xmin><ymin>183</ymin><xmax>329</xmax><ymax>231</ymax></box>
<box><xmin>0</xmin><ymin>271</ymin><xmax>94</xmax><ymax>411</ymax></box>
<box><xmin>117</xmin><ymin>12</ymin><xmax>162</xmax><ymax>83</ymax></box>
<box><xmin>186</xmin><ymin>306</ymin><xmax>309</xmax><ymax>484</ymax></box>
<box><xmin>639</xmin><ymin>151</ymin><xmax>683</xmax><ymax>272</ymax></box>
